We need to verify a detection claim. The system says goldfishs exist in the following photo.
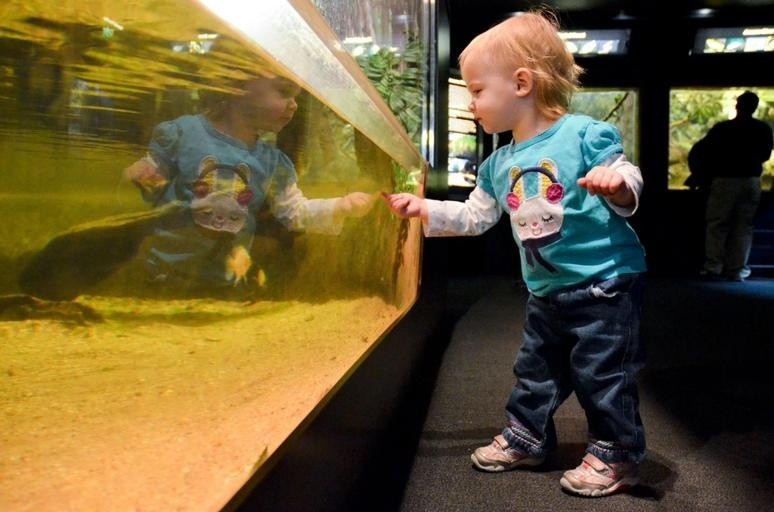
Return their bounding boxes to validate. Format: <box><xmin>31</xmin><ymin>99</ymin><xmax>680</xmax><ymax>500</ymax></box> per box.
<box><xmin>145</xmin><ymin>274</ymin><xmax>167</xmax><ymax>286</ymax></box>
<box><xmin>224</xmin><ymin>245</ymin><xmax>252</xmax><ymax>288</ymax></box>
<box><xmin>252</xmin><ymin>268</ymin><xmax>266</xmax><ymax>289</ymax></box>
<box><xmin>140</xmin><ymin>173</ymin><xmax>168</xmax><ymax>189</ymax></box>
<box><xmin>294</xmin><ymin>162</ymin><xmax>306</xmax><ymax>176</ymax></box>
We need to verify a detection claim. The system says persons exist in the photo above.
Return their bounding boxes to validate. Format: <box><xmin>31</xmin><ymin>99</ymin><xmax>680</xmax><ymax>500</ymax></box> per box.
<box><xmin>122</xmin><ymin>71</ymin><xmax>385</xmax><ymax>299</ymax></box>
<box><xmin>382</xmin><ymin>4</ymin><xmax>648</xmax><ymax>500</ymax></box>
<box><xmin>688</xmin><ymin>91</ymin><xmax>774</xmax><ymax>282</ymax></box>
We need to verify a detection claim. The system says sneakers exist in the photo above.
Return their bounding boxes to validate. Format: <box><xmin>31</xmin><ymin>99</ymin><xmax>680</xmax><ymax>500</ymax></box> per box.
<box><xmin>696</xmin><ymin>268</ymin><xmax>751</xmax><ymax>282</ymax></box>
<box><xmin>470</xmin><ymin>432</ymin><xmax>546</xmax><ymax>473</ymax></box>
<box><xmin>560</xmin><ymin>452</ymin><xmax>640</xmax><ymax>497</ymax></box>
